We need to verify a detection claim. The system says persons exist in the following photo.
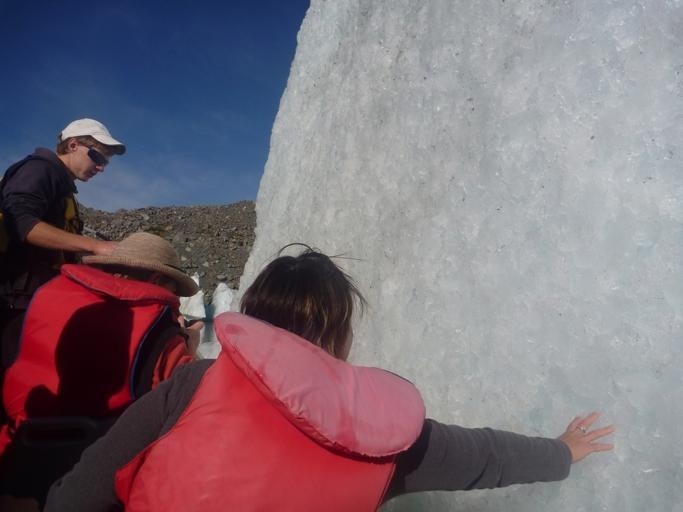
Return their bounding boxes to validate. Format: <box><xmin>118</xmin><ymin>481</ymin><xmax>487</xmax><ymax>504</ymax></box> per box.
<box><xmin>0</xmin><ymin>231</ymin><xmax>204</xmax><ymax>510</ymax></box>
<box><xmin>1</xmin><ymin>120</ymin><xmax>126</xmax><ymax>401</ymax></box>
<box><xmin>43</xmin><ymin>245</ymin><xmax>617</xmax><ymax>511</ymax></box>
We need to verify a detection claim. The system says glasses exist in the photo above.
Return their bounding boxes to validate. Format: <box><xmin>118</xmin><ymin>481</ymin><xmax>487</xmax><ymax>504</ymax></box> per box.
<box><xmin>77</xmin><ymin>139</ymin><xmax>109</xmax><ymax>166</ymax></box>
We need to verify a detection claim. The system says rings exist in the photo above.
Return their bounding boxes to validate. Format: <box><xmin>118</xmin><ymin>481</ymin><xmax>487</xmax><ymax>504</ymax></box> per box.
<box><xmin>577</xmin><ymin>425</ymin><xmax>586</xmax><ymax>433</ymax></box>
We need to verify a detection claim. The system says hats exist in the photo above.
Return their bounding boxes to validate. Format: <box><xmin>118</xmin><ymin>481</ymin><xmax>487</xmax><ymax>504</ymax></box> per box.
<box><xmin>60</xmin><ymin>118</ymin><xmax>125</xmax><ymax>155</ymax></box>
<box><xmin>81</xmin><ymin>230</ymin><xmax>200</xmax><ymax>297</ymax></box>
